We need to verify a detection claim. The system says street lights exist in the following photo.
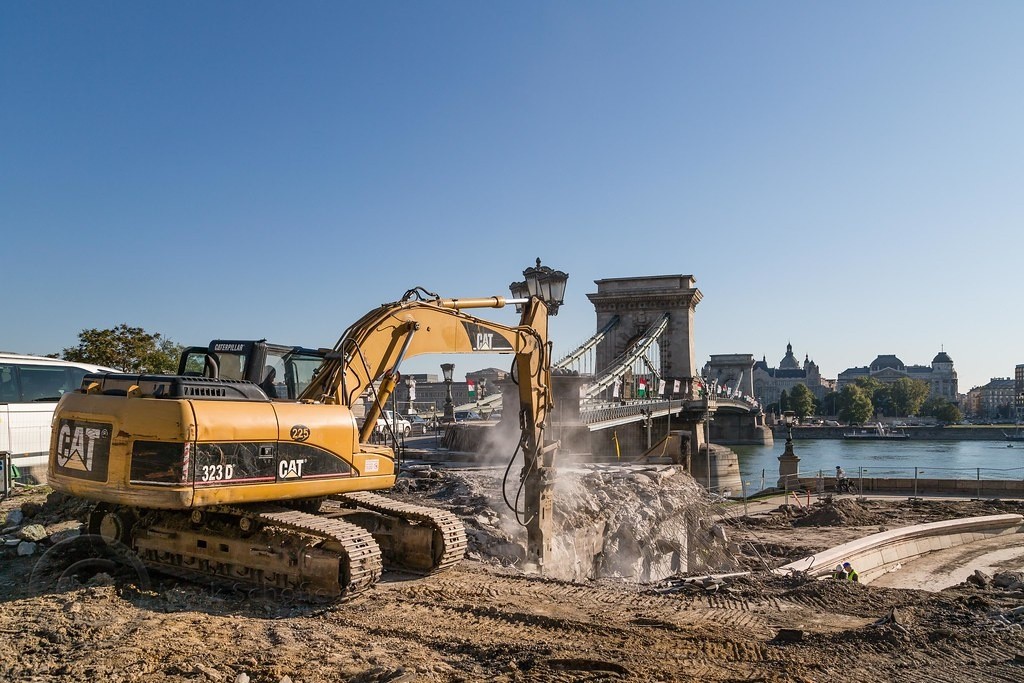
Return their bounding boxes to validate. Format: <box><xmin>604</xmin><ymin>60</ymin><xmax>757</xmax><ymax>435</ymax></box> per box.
<box><xmin>781</xmin><ymin>409</ymin><xmax>797</xmax><ymax>456</ymax></box>
<box><xmin>477</xmin><ymin>376</ymin><xmax>488</xmax><ymax>401</ymax></box>
<box><xmin>440</xmin><ymin>363</ymin><xmax>456</xmax><ymax>406</ymax></box>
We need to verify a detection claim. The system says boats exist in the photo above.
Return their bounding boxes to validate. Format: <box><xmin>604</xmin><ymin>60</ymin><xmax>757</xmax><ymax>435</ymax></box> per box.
<box><xmin>843</xmin><ymin>421</ymin><xmax>911</xmax><ymax>441</ymax></box>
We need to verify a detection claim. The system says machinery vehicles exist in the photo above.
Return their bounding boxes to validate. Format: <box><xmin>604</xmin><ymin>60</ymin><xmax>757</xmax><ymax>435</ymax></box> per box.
<box><xmin>46</xmin><ymin>293</ymin><xmax>556</xmax><ymax>608</ymax></box>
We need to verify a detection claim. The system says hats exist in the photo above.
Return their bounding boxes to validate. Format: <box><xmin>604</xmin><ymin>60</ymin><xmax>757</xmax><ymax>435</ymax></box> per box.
<box><xmin>843</xmin><ymin>562</ymin><xmax>850</xmax><ymax>569</ymax></box>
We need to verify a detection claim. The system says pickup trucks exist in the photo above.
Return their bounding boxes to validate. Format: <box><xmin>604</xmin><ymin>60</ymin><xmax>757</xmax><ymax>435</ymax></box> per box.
<box><xmin>355</xmin><ymin>410</ymin><xmax>412</xmax><ymax>438</ymax></box>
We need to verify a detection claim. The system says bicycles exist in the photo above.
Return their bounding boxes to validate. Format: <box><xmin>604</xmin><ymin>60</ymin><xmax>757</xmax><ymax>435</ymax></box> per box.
<box><xmin>831</xmin><ymin>477</ymin><xmax>859</xmax><ymax>495</ymax></box>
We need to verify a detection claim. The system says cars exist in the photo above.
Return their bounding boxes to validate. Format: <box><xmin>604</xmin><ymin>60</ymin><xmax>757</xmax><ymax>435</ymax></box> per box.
<box><xmin>400</xmin><ymin>408</ymin><xmax>502</xmax><ymax>436</ymax></box>
<box><xmin>892</xmin><ymin>420</ymin><xmax>907</xmax><ymax>426</ymax></box>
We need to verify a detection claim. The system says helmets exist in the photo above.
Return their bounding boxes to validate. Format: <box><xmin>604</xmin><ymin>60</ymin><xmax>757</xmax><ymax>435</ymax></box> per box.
<box><xmin>836</xmin><ymin>565</ymin><xmax>843</xmax><ymax>572</ymax></box>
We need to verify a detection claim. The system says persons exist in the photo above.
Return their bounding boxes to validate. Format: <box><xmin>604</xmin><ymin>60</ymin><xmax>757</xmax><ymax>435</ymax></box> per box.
<box><xmin>836</xmin><ymin>466</ymin><xmax>845</xmax><ymax>495</ymax></box>
<box><xmin>263</xmin><ymin>365</ymin><xmax>279</xmax><ymax>398</ymax></box>
<box><xmin>843</xmin><ymin>562</ymin><xmax>858</xmax><ymax>581</ymax></box>
<box><xmin>832</xmin><ymin>565</ymin><xmax>846</xmax><ymax>580</ymax></box>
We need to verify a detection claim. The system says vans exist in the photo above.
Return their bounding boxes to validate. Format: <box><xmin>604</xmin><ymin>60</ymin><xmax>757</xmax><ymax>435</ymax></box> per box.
<box><xmin>826</xmin><ymin>421</ymin><xmax>840</xmax><ymax>426</ymax></box>
<box><xmin>0</xmin><ymin>352</ymin><xmax>125</xmax><ymax>480</ymax></box>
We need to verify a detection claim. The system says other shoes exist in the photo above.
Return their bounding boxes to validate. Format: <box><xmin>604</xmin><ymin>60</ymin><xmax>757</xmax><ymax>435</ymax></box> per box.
<box><xmin>837</xmin><ymin>492</ymin><xmax>843</xmax><ymax>495</ymax></box>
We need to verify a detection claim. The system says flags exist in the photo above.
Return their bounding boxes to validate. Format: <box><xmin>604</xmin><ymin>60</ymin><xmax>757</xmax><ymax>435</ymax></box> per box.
<box><xmin>685</xmin><ymin>381</ymin><xmax>689</xmax><ymax>394</ymax></box>
<box><xmin>673</xmin><ymin>380</ymin><xmax>681</xmax><ymax>393</ymax></box>
<box><xmin>466</xmin><ymin>376</ymin><xmax>476</xmax><ymax>397</ymax></box>
<box><xmin>613</xmin><ymin>379</ymin><xmax>623</xmax><ymax>398</ymax></box>
<box><xmin>637</xmin><ymin>377</ymin><xmax>647</xmax><ymax>396</ymax></box>
<box><xmin>698</xmin><ymin>382</ymin><xmax>758</xmax><ymax>407</ymax></box>
<box><xmin>658</xmin><ymin>379</ymin><xmax>666</xmax><ymax>394</ymax></box>
